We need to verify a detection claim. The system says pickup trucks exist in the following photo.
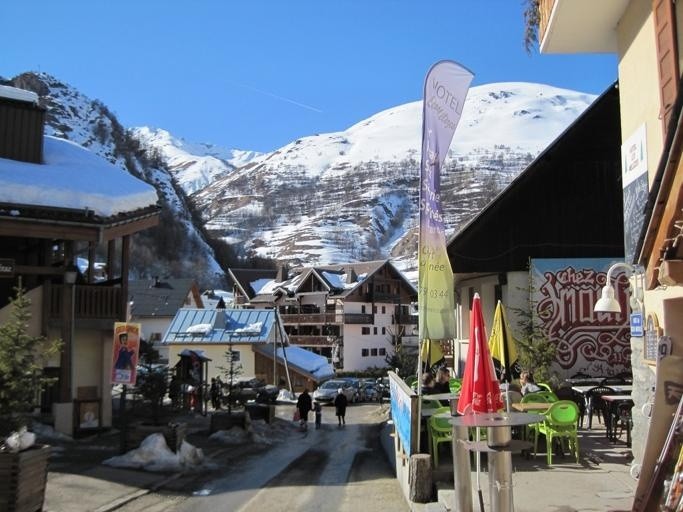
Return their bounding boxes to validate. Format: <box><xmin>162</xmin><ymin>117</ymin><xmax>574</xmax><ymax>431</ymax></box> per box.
<box><xmin>231</xmin><ymin>377</ymin><xmax>279</xmax><ymax>403</ymax></box>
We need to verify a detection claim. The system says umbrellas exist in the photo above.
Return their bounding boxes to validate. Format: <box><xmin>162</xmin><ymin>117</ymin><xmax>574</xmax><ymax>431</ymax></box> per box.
<box><xmin>488</xmin><ymin>298</ymin><xmax>521</xmax><ymax>372</ymax></box>
<box><xmin>457</xmin><ymin>291</ymin><xmax>504</xmax><ymax>512</ymax></box>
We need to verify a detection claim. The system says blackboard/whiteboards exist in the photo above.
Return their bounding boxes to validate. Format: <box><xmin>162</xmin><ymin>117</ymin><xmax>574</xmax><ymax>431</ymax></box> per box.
<box><xmin>641</xmin><ymin>312</ymin><xmax>661</xmax><ymax>364</ymax></box>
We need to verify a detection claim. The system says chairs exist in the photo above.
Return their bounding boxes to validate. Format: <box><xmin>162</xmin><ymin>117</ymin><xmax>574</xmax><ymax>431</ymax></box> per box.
<box><xmin>405</xmin><ymin>372</ymin><xmax>632</xmax><ymax>469</ymax></box>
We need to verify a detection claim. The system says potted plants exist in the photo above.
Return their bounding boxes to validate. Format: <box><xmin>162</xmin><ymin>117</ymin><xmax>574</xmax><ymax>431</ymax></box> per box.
<box><xmin>0</xmin><ymin>275</ymin><xmax>66</xmax><ymax>512</ymax></box>
<box><xmin>244</xmin><ymin>379</ymin><xmax>275</xmax><ymax>423</ymax></box>
<box><xmin>125</xmin><ymin>339</ymin><xmax>183</xmax><ymax>454</ymax></box>
<box><xmin>211</xmin><ymin>335</ymin><xmax>245</xmax><ymax>432</ymax></box>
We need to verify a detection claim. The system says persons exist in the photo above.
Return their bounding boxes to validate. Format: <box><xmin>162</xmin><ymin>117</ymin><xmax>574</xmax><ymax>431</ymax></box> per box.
<box><xmin>435</xmin><ymin>367</ymin><xmax>453</xmax><ymax>393</ymax></box>
<box><xmin>215</xmin><ymin>375</ymin><xmax>223</xmax><ymax>405</ymax></box>
<box><xmin>494</xmin><ymin>367</ymin><xmax>508</xmax><ymax>391</ymax></box>
<box><xmin>411</xmin><ymin>371</ymin><xmax>451</xmax><ymax>446</ymax></box>
<box><xmin>295</xmin><ymin>389</ymin><xmax>312</xmax><ymax>427</ymax></box>
<box><xmin>167</xmin><ymin>374</ymin><xmax>176</xmax><ymax>402</ymax></box>
<box><xmin>209</xmin><ymin>377</ymin><xmax>218</xmax><ymax>409</ymax></box>
<box><xmin>311</xmin><ymin>401</ymin><xmax>322</xmax><ymax>429</ymax></box>
<box><xmin>112</xmin><ymin>331</ymin><xmax>135</xmax><ymax>376</ymax></box>
<box><xmin>517</xmin><ymin>369</ymin><xmax>543</xmax><ymax>397</ymax></box>
<box><xmin>334</xmin><ymin>387</ymin><xmax>346</xmax><ymax>426</ymax></box>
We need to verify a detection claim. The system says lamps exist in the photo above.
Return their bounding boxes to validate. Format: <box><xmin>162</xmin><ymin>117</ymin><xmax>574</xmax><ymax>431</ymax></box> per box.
<box><xmin>594</xmin><ymin>263</ymin><xmax>645</xmax><ymax>320</ymax></box>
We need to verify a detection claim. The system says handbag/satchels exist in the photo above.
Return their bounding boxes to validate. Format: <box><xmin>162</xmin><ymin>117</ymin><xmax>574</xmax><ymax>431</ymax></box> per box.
<box><xmin>293</xmin><ymin>413</ymin><xmax>301</xmax><ymax>421</ymax></box>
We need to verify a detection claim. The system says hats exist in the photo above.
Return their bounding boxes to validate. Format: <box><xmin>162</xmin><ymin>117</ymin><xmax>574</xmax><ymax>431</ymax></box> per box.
<box><xmin>337</xmin><ymin>388</ymin><xmax>343</xmax><ymax>394</ymax></box>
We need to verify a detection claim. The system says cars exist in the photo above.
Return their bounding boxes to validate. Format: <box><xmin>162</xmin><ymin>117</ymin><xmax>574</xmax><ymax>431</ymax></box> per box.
<box><xmin>311</xmin><ymin>377</ymin><xmax>390</xmax><ymax>406</ymax></box>
